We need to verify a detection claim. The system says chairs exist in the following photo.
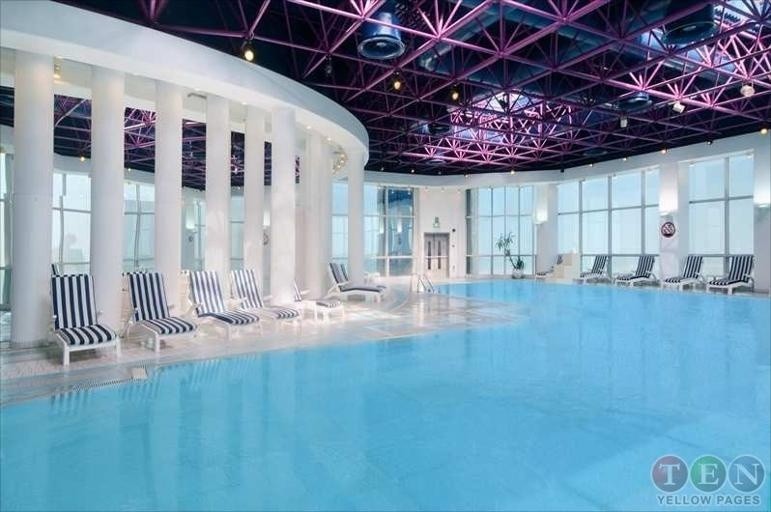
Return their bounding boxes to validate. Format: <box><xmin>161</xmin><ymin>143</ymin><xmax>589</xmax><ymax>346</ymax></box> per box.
<box><xmin>535</xmin><ymin>253</ymin><xmax>565</xmax><ymax>279</ymax></box>
<box><xmin>704</xmin><ymin>254</ymin><xmax>756</xmax><ymax>294</ymax></box>
<box><xmin>571</xmin><ymin>256</ymin><xmax>612</xmax><ymax>285</ymax></box>
<box><xmin>660</xmin><ymin>256</ymin><xmax>705</xmax><ymax>291</ymax></box>
<box><xmin>49</xmin><ymin>268</ymin><xmax>344</xmax><ymax>367</ymax></box>
<box><xmin>613</xmin><ymin>254</ymin><xmax>657</xmax><ymax>286</ymax></box>
<box><xmin>319</xmin><ymin>263</ymin><xmax>390</xmax><ymax>303</ymax></box>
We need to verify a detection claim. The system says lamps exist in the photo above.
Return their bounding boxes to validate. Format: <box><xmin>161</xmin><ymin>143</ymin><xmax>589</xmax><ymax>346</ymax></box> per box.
<box><xmin>620</xmin><ymin>118</ymin><xmax>627</xmax><ymax>128</ymax></box>
<box><xmin>449</xmin><ymin>83</ymin><xmax>458</xmax><ymax>101</ymax></box>
<box><xmin>660</xmin><ymin>0</ymin><xmax>720</xmax><ymax>44</ymax></box>
<box><xmin>392</xmin><ymin>72</ymin><xmax>401</xmax><ymax>90</ymax></box>
<box><xmin>672</xmin><ymin>100</ymin><xmax>686</xmax><ymax>113</ymax></box>
<box><xmin>242</xmin><ymin>38</ymin><xmax>256</xmax><ymax>61</ymax></box>
<box><xmin>354</xmin><ymin>0</ymin><xmax>404</xmax><ymax>59</ymax></box>
<box><xmin>739</xmin><ymin>77</ymin><xmax>755</xmax><ymax>96</ymax></box>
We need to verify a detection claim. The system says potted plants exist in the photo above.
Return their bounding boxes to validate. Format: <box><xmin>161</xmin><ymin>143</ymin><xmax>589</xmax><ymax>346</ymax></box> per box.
<box><xmin>496</xmin><ymin>230</ymin><xmax>525</xmax><ymax>279</ymax></box>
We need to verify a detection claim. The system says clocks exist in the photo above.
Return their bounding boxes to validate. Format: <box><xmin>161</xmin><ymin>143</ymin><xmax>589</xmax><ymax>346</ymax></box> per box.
<box><xmin>660</xmin><ymin>222</ymin><xmax>676</xmax><ymax>238</ymax></box>
